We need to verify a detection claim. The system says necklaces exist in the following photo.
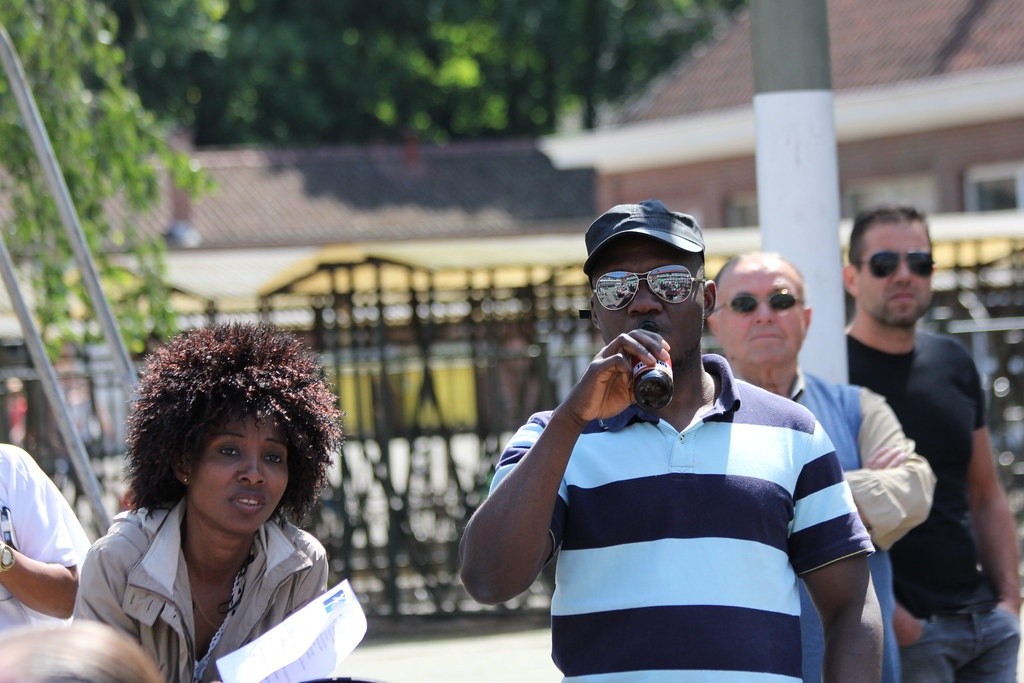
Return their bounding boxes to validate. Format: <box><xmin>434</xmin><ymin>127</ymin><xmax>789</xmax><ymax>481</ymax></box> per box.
<box><xmin>193</xmin><ymin>586</ymin><xmax>225</xmax><ymax>631</ymax></box>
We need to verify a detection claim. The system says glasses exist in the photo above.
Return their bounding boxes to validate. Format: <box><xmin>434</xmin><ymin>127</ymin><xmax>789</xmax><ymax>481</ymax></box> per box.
<box><xmin>714</xmin><ymin>293</ymin><xmax>805</xmax><ymax>313</ymax></box>
<box><xmin>592</xmin><ymin>265</ymin><xmax>706</xmax><ymax>310</ymax></box>
<box><xmin>861</xmin><ymin>251</ymin><xmax>936</xmax><ymax>279</ymax></box>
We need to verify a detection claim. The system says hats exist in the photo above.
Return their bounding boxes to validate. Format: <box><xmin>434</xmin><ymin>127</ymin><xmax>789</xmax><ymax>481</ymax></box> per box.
<box><xmin>583</xmin><ymin>198</ymin><xmax>705</xmax><ymax>292</ymax></box>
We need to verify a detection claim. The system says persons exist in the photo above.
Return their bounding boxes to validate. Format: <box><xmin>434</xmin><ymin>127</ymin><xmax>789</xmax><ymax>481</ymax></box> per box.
<box><xmin>707</xmin><ymin>252</ymin><xmax>935</xmax><ymax>682</ymax></box>
<box><xmin>845</xmin><ymin>206</ymin><xmax>1024</xmax><ymax>683</ymax></box>
<box><xmin>0</xmin><ymin>443</ymin><xmax>91</xmax><ymax>630</ymax></box>
<box><xmin>1</xmin><ymin>619</ymin><xmax>161</xmax><ymax>682</ymax></box>
<box><xmin>74</xmin><ymin>323</ymin><xmax>347</xmax><ymax>683</ymax></box>
<box><xmin>456</xmin><ymin>198</ymin><xmax>884</xmax><ymax>682</ymax></box>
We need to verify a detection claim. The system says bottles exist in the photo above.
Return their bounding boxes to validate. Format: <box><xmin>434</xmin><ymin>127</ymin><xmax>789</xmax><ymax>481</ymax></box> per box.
<box><xmin>631</xmin><ymin>325</ymin><xmax>674</xmax><ymax>412</ymax></box>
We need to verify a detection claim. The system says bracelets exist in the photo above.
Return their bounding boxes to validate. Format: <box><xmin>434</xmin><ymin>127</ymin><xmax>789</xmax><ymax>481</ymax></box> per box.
<box><xmin>0</xmin><ymin>543</ymin><xmax>16</xmax><ymax>571</ymax></box>
